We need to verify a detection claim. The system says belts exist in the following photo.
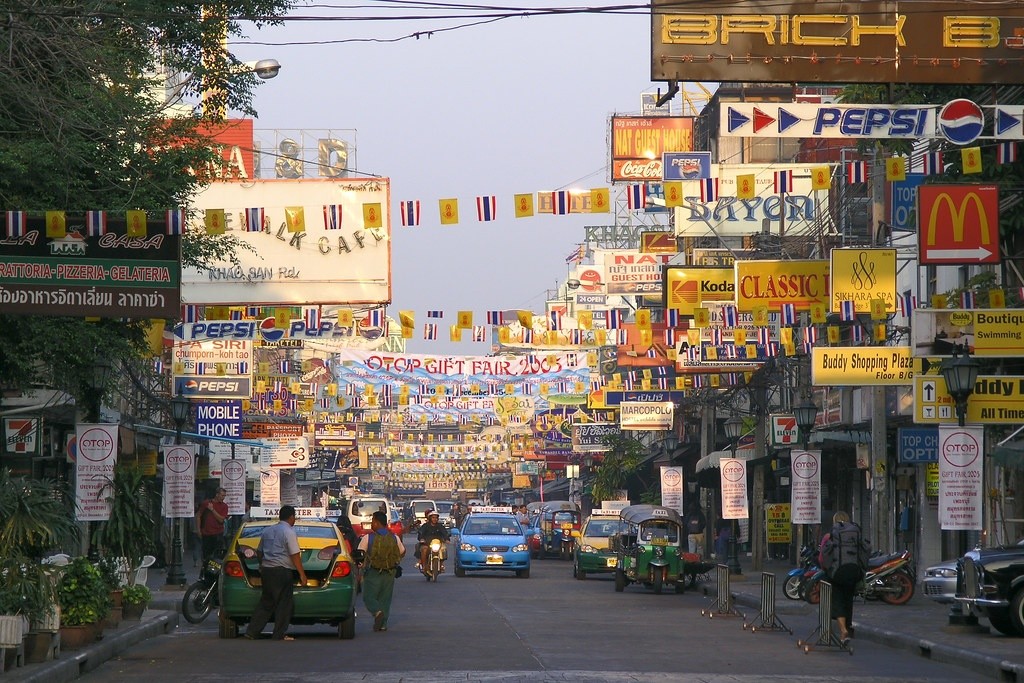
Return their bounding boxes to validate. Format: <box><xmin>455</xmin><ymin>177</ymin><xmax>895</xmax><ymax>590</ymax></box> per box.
<box><xmin>371</xmin><ymin>566</ymin><xmax>395</xmax><ymax>572</ymax></box>
<box><xmin>688</xmin><ymin>532</ymin><xmax>699</xmax><ymax>534</ymax></box>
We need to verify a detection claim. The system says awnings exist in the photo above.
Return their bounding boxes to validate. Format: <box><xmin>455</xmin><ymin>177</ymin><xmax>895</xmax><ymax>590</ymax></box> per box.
<box><xmin>695</xmin><ymin>447</ymin><xmax>768</xmax><ymax>473</ymax></box>
<box><xmin>133</xmin><ymin>424</ymin><xmax>271</xmax><ymax>459</ymax></box>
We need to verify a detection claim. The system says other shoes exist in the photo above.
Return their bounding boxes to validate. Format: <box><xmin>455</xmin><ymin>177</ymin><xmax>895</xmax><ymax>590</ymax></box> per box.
<box><xmin>373</xmin><ymin>611</ymin><xmax>384</xmax><ymax>631</ymax></box>
<box><xmin>244</xmin><ymin>633</ymin><xmax>255</xmax><ymax>640</ymax></box>
<box><xmin>847</xmin><ymin>626</ymin><xmax>855</xmax><ymax>637</ymax></box>
<box><xmin>442</xmin><ymin>565</ymin><xmax>445</xmax><ymax>571</ymax></box>
<box><xmin>414</xmin><ymin>562</ymin><xmax>420</xmax><ymax>569</ymax></box>
<box><xmin>283</xmin><ymin>634</ymin><xmax>295</xmax><ymax>641</ymax></box>
<box><xmin>379</xmin><ymin>627</ymin><xmax>387</xmax><ymax>631</ymax></box>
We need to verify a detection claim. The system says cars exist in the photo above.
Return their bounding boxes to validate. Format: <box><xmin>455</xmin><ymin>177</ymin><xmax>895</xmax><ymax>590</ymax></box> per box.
<box><xmin>389</xmin><ymin>500</ymin><xmax>456</xmax><ymax>529</ymax></box>
<box><xmin>571</xmin><ymin>515</ymin><xmax>637</xmax><ymax>580</ymax></box>
<box><xmin>954</xmin><ymin>539</ymin><xmax>1024</xmax><ymax>636</ymax></box>
<box><xmin>450</xmin><ymin>506</ymin><xmax>531</xmax><ymax>579</ymax></box>
<box><xmin>923</xmin><ymin>558</ymin><xmax>962</xmax><ymax>602</ymax></box>
<box><xmin>217</xmin><ymin>505</ymin><xmax>358</xmax><ymax>639</ymax></box>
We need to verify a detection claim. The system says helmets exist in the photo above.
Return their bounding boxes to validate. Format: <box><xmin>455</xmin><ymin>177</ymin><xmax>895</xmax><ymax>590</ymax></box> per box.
<box><xmin>427</xmin><ymin>511</ymin><xmax>440</xmax><ymax>518</ymax></box>
<box><xmin>424</xmin><ymin>507</ymin><xmax>433</xmax><ymax>518</ymax></box>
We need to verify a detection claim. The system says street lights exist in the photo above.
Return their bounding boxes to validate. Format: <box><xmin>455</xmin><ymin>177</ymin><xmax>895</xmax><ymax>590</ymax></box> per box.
<box><xmin>84</xmin><ymin>348</ymin><xmax>112</xmax><ymax>564</ymax></box>
<box><xmin>937</xmin><ymin>339</ymin><xmax>979</xmax><ymax>625</ymax></box>
<box><xmin>724</xmin><ymin>417</ymin><xmax>745</xmax><ymax>575</ymax></box>
<box><xmin>793</xmin><ymin>398</ymin><xmax>819</xmax><ymax>568</ymax></box>
<box><xmin>166</xmin><ymin>380</ymin><xmax>190</xmax><ymax>585</ymax></box>
<box><xmin>662</xmin><ymin>433</ymin><xmax>679</xmax><ymax>466</ymax></box>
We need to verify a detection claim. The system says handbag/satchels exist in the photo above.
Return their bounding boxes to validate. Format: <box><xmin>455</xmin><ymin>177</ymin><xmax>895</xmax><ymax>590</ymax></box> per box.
<box><xmin>833</xmin><ymin>563</ymin><xmax>865</xmax><ymax>592</ymax></box>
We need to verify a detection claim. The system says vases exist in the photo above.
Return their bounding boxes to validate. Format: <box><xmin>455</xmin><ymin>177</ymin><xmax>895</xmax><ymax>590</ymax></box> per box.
<box><xmin>684</xmin><ymin>562</ymin><xmax>716</xmax><ymax>591</ymax></box>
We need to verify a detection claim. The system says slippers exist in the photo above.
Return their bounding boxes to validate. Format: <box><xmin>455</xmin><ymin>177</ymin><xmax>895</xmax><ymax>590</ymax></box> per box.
<box><xmin>841</xmin><ymin>637</ymin><xmax>851</xmax><ymax>650</ymax></box>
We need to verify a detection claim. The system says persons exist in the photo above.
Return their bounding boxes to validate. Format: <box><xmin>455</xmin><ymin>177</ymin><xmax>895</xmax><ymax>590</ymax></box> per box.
<box><xmin>817</xmin><ymin>511</ymin><xmax>872</xmax><ymax>650</ymax></box>
<box><xmin>244</xmin><ymin>505</ymin><xmax>307</xmax><ymax>642</ymax></box>
<box><xmin>413</xmin><ymin>508</ymin><xmax>450</xmax><ymax>573</ymax></box>
<box><xmin>335</xmin><ymin>511</ymin><xmax>406</xmax><ymax>633</ymax></box>
<box><xmin>713</xmin><ymin>512</ymin><xmax>741</xmax><ymax>557</ymax></box>
<box><xmin>450</xmin><ymin>500</ymin><xmax>530</xmax><ymax>533</ymax></box>
<box><xmin>189</xmin><ymin>487</ymin><xmax>228</xmax><ymax>567</ymax></box>
<box><xmin>683</xmin><ymin>500</ymin><xmax>707</xmax><ymax>561</ymax></box>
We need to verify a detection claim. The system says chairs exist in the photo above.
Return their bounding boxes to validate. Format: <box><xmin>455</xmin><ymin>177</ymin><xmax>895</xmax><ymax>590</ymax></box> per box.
<box><xmin>38</xmin><ymin>552</ymin><xmax>157</xmax><ymax>611</ymax></box>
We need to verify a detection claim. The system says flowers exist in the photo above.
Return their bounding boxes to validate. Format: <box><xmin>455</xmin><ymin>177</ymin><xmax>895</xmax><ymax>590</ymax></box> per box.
<box><xmin>683</xmin><ymin>551</ymin><xmax>706</xmax><ymax>564</ymax></box>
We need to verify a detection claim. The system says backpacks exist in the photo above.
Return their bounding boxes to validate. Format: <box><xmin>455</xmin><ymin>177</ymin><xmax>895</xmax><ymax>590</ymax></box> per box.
<box><xmin>366</xmin><ymin>529</ymin><xmax>401</xmax><ymax>571</ymax></box>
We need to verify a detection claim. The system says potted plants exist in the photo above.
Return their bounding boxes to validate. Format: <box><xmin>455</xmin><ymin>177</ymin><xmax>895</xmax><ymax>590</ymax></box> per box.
<box><xmin>0</xmin><ymin>463</ymin><xmax>152</xmax><ymax>669</ymax></box>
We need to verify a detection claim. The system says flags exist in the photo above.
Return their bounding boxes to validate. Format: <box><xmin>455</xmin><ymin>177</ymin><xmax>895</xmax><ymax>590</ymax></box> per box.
<box><xmin>0</xmin><ymin>140</ymin><xmax>1023</xmax><ymax>414</ymax></box>
<box><xmin>74</xmin><ymin>422</ymin><xmax>118</xmax><ymax>523</ymax></box>
<box><xmin>220</xmin><ymin>459</ymin><xmax>247</xmax><ymax>515</ymax></box>
<box><xmin>719</xmin><ymin>457</ymin><xmax>751</xmax><ymax>519</ymax></box>
<box><xmin>935</xmin><ymin>423</ymin><xmax>984</xmax><ymax>534</ymax></box>
<box><xmin>788</xmin><ymin>448</ymin><xmax>825</xmax><ymax>524</ymax></box>
<box><xmin>660</xmin><ymin>464</ymin><xmax>685</xmax><ymax>515</ymax></box>
<box><xmin>259</xmin><ymin>468</ymin><xmax>281</xmax><ymax>508</ymax></box>
<box><xmin>161</xmin><ymin>445</ymin><xmax>195</xmax><ymax>519</ymax></box>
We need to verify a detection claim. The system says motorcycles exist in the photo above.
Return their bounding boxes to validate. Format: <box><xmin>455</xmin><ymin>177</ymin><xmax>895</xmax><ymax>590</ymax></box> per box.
<box><xmin>349</xmin><ymin>497</ymin><xmax>392</xmax><ymax>536</ymax></box>
<box><xmin>614</xmin><ymin>504</ymin><xmax>685</xmax><ymax>595</ymax></box>
<box><xmin>526</xmin><ymin>502</ymin><xmax>581</xmax><ymax>561</ymax></box>
<box><xmin>782</xmin><ymin>526</ymin><xmax>916</xmax><ymax>605</ymax></box>
<box><xmin>417</xmin><ymin>530</ymin><xmax>448</xmax><ymax>584</ymax></box>
<box><xmin>182</xmin><ymin>534</ymin><xmax>232</xmax><ymax>625</ymax></box>
